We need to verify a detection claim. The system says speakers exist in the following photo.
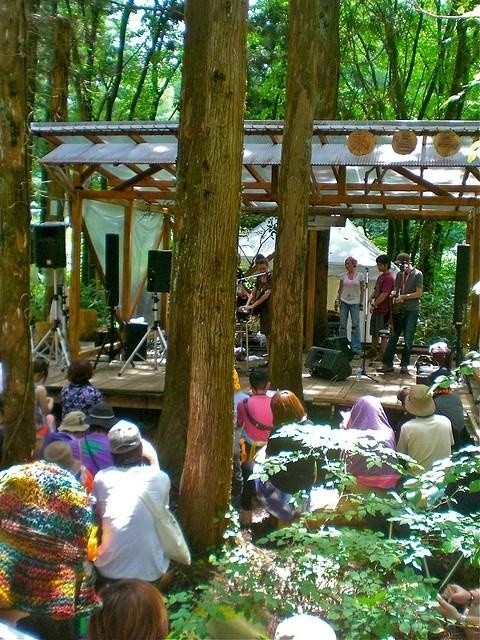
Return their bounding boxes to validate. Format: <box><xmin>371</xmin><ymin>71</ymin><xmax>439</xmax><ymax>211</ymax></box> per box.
<box><xmin>454</xmin><ymin>243</ymin><xmax>470</xmax><ymax>305</ymax></box>
<box><xmin>105</xmin><ymin>234</ymin><xmax>120</xmax><ymax>306</ymax></box>
<box><xmin>147</xmin><ymin>249</ymin><xmax>171</xmax><ymax>293</ymax></box>
<box><xmin>33</xmin><ymin>221</ymin><xmax>67</xmax><ymax>268</ymax></box>
<box><xmin>304</xmin><ymin>346</ymin><xmax>352</xmax><ymax>381</ymax></box>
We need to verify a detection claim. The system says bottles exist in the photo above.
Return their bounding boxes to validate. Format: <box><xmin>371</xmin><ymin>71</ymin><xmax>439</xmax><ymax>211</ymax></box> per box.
<box><xmin>354</xmin><ymin>358</ymin><xmax>362</xmax><ymax>382</ymax></box>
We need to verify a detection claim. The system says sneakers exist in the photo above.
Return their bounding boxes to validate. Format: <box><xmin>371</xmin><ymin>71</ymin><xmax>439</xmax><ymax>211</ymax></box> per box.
<box><xmin>375</xmin><ymin>353</ymin><xmax>383</xmax><ymax>361</ymax></box>
<box><xmin>361</xmin><ymin>350</ymin><xmax>376</xmax><ymax>357</ymax></box>
<box><xmin>376</xmin><ymin>365</ymin><xmax>393</xmax><ymax>372</ymax></box>
<box><xmin>353</xmin><ymin>353</ymin><xmax>360</xmax><ymax>360</ymax></box>
<box><xmin>400</xmin><ymin>366</ymin><xmax>407</xmax><ymax>373</ymax></box>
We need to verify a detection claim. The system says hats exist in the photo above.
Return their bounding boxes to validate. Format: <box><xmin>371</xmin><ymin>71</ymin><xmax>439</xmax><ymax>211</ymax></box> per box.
<box><xmin>394</xmin><ymin>253</ymin><xmax>410</xmax><ymax>265</ymax></box>
<box><xmin>57</xmin><ymin>412</ymin><xmax>90</xmax><ymax>432</ymax></box>
<box><xmin>0</xmin><ymin>460</ymin><xmax>104</xmax><ymax>621</ymax></box>
<box><xmin>43</xmin><ymin>441</ymin><xmax>83</xmax><ymax>475</ymax></box>
<box><xmin>86</xmin><ymin>403</ymin><xmax>118</xmax><ymax>429</ymax></box>
<box><xmin>405</xmin><ymin>384</ymin><xmax>437</xmax><ymax>417</ymax></box>
<box><xmin>273</xmin><ymin>614</ymin><xmax>337</xmax><ymax>640</ymax></box>
<box><xmin>108</xmin><ymin>419</ymin><xmax>142</xmax><ymax>455</ymax></box>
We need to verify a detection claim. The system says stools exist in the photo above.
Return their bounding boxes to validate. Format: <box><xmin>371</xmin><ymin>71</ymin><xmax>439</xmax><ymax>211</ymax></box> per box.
<box><xmin>369</xmin><ymin>328</ymin><xmax>403</xmax><ymax>368</ymax></box>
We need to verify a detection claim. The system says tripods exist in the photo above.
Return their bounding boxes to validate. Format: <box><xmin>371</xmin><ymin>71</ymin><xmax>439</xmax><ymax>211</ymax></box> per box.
<box><xmin>119</xmin><ymin>294</ymin><xmax>168</xmax><ymax>384</ymax></box>
<box><xmin>35</xmin><ymin>270</ymin><xmax>75</xmax><ymax>368</ymax></box>
<box><xmin>95</xmin><ymin>308</ymin><xmax>135</xmax><ymax>372</ymax></box>
<box><xmin>438</xmin><ymin>323</ymin><xmax>472</xmax><ymax>395</ymax></box>
<box><xmin>349</xmin><ymin>288</ymin><xmax>380</xmax><ymax>383</ymax></box>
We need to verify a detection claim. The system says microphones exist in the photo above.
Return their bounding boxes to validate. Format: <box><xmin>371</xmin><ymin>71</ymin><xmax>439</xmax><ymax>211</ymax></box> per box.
<box><xmin>364</xmin><ymin>265</ymin><xmax>370</xmax><ymax>286</ymax></box>
